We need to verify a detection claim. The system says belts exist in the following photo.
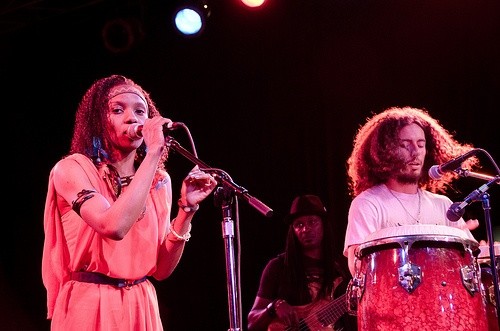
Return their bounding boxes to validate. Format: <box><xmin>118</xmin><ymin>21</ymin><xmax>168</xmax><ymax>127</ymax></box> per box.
<box><xmin>70</xmin><ymin>271</ymin><xmax>148</xmax><ymax>288</ymax></box>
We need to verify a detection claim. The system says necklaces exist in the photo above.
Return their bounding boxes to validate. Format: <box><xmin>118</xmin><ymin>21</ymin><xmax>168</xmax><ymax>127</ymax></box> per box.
<box><xmin>381</xmin><ymin>178</ymin><xmax>424</xmax><ymax>227</ymax></box>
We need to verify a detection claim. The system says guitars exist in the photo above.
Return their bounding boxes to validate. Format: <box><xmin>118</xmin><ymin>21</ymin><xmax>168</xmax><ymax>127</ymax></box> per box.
<box><xmin>266</xmin><ymin>277</ymin><xmax>347</xmax><ymax>331</ymax></box>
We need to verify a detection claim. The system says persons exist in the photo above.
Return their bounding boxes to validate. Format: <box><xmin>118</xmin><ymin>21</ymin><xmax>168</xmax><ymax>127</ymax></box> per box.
<box><xmin>243</xmin><ymin>194</ymin><xmax>359</xmax><ymax>331</ymax></box>
<box><xmin>40</xmin><ymin>75</ymin><xmax>216</xmax><ymax>330</ymax></box>
<box><xmin>342</xmin><ymin>108</ymin><xmax>482</xmax><ymax>278</ymax></box>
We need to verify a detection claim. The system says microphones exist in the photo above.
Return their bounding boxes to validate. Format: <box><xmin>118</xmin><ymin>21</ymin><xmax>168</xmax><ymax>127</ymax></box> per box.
<box><xmin>446</xmin><ymin>178</ymin><xmax>498</xmax><ymax>222</ymax></box>
<box><xmin>126</xmin><ymin>122</ymin><xmax>184</xmax><ymax>140</ymax></box>
<box><xmin>429</xmin><ymin>148</ymin><xmax>482</xmax><ymax>180</ymax></box>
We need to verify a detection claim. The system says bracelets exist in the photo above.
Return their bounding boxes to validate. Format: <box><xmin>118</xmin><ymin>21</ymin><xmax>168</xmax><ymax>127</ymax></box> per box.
<box><xmin>266</xmin><ymin>297</ymin><xmax>280</xmax><ymax>319</ymax></box>
<box><xmin>167</xmin><ymin>217</ymin><xmax>192</xmax><ymax>242</ymax></box>
<box><xmin>177</xmin><ymin>196</ymin><xmax>200</xmax><ymax>213</ymax></box>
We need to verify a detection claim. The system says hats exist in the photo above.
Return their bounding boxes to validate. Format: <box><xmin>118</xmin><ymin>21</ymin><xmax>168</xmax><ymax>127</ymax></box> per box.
<box><xmin>289</xmin><ymin>195</ymin><xmax>328</xmax><ymax>220</ymax></box>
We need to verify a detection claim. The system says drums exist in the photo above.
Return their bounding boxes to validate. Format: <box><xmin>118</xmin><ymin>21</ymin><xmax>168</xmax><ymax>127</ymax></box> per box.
<box><xmin>345</xmin><ymin>224</ymin><xmax>500</xmax><ymax>331</ymax></box>
